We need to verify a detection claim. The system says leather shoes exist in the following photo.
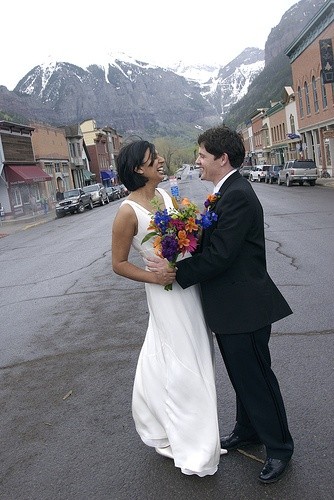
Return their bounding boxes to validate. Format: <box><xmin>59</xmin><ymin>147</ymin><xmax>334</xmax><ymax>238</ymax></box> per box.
<box><xmin>220</xmin><ymin>428</ymin><xmax>261</xmax><ymax>451</ymax></box>
<box><xmin>258</xmin><ymin>455</ymin><xmax>291</xmax><ymax>483</ymax></box>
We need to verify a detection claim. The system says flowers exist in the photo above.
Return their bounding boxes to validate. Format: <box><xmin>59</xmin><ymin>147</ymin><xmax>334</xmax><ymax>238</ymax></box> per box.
<box><xmin>196</xmin><ymin>191</ymin><xmax>221</xmax><ymax>229</ymax></box>
<box><xmin>141</xmin><ymin>196</ymin><xmax>201</xmax><ymax>292</ymax></box>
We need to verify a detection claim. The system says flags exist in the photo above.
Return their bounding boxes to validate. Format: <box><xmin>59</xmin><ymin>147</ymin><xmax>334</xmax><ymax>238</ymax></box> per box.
<box><xmin>287</xmin><ymin>133</ymin><xmax>300</xmax><ymax>139</ymax></box>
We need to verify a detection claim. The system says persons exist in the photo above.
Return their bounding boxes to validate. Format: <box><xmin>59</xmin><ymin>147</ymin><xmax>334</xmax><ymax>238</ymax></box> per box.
<box><xmin>55</xmin><ymin>187</ymin><xmax>65</xmax><ymax>202</ymax></box>
<box><xmin>110</xmin><ymin>138</ymin><xmax>228</xmax><ymax>476</ymax></box>
<box><xmin>144</xmin><ymin>125</ymin><xmax>297</xmax><ymax>484</ymax></box>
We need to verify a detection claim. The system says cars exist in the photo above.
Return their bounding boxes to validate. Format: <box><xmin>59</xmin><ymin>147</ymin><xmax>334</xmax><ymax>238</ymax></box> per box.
<box><xmin>161</xmin><ymin>164</ymin><xmax>201</xmax><ymax>182</ymax></box>
<box><xmin>104</xmin><ymin>186</ymin><xmax>121</xmax><ymax>201</ymax></box>
<box><xmin>114</xmin><ymin>184</ymin><xmax>130</xmax><ymax>198</ymax></box>
<box><xmin>82</xmin><ymin>182</ymin><xmax>109</xmax><ymax>207</ymax></box>
<box><xmin>239</xmin><ymin>166</ymin><xmax>252</xmax><ymax>176</ymax></box>
<box><xmin>249</xmin><ymin>165</ymin><xmax>271</xmax><ymax>182</ymax></box>
<box><xmin>264</xmin><ymin>165</ymin><xmax>281</xmax><ymax>184</ymax></box>
<box><xmin>55</xmin><ymin>188</ymin><xmax>94</xmax><ymax>218</ymax></box>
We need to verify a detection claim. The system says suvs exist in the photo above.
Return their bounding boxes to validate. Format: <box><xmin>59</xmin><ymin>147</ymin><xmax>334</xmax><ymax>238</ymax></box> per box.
<box><xmin>276</xmin><ymin>159</ymin><xmax>320</xmax><ymax>187</ymax></box>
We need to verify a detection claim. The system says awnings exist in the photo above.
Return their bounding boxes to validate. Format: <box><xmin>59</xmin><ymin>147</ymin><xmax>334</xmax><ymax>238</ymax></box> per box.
<box><xmin>3</xmin><ymin>163</ymin><xmax>52</xmax><ymax>188</ymax></box>
